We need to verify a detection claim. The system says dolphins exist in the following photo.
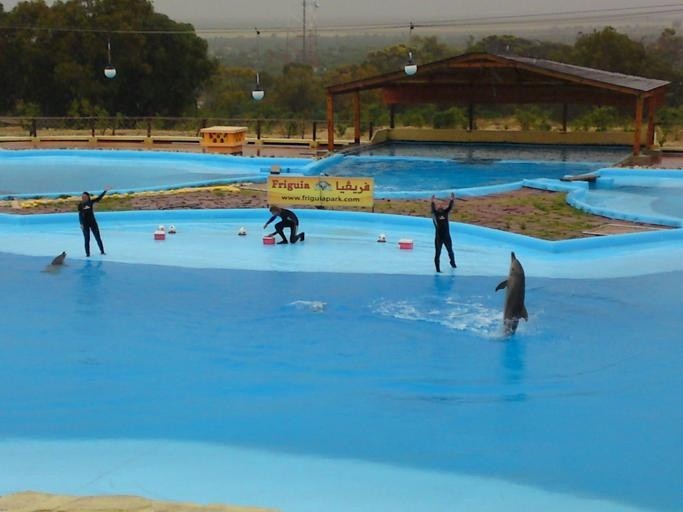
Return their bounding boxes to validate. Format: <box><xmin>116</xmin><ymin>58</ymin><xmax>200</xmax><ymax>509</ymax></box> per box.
<box><xmin>44</xmin><ymin>252</ymin><xmax>67</xmax><ymax>272</ymax></box>
<box><xmin>495</xmin><ymin>251</ymin><xmax>528</xmax><ymax>337</ymax></box>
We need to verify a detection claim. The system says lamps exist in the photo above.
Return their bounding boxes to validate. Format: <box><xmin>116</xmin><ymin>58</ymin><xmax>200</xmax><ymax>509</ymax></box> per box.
<box><xmin>103</xmin><ymin>33</ymin><xmax>117</xmax><ymax>79</ymax></box>
<box><xmin>251</xmin><ymin>31</ymin><xmax>265</xmax><ymax>101</ymax></box>
<box><xmin>404</xmin><ymin>28</ymin><xmax>418</xmax><ymax>76</ymax></box>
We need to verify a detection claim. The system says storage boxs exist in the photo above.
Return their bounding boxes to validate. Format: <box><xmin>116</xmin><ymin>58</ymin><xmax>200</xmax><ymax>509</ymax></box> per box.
<box><xmin>399</xmin><ymin>240</ymin><xmax>412</xmax><ymax>249</ymax></box>
<box><xmin>154</xmin><ymin>231</ymin><xmax>166</xmax><ymax>240</ymax></box>
<box><xmin>263</xmin><ymin>237</ymin><xmax>275</xmax><ymax>244</ymax></box>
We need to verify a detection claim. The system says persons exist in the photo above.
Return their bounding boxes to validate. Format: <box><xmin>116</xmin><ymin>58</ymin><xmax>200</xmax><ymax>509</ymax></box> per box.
<box><xmin>430</xmin><ymin>191</ymin><xmax>457</xmax><ymax>272</ymax></box>
<box><xmin>263</xmin><ymin>207</ymin><xmax>305</xmax><ymax>245</ymax></box>
<box><xmin>77</xmin><ymin>184</ymin><xmax>112</xmax><ymax>257</ymax></box>
